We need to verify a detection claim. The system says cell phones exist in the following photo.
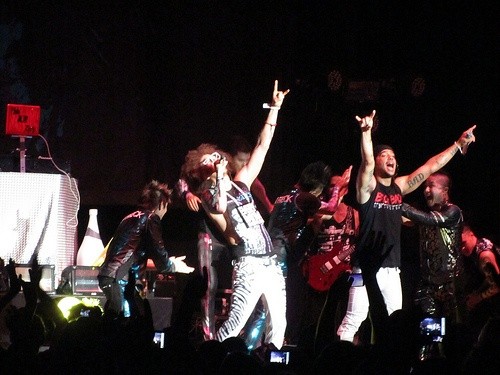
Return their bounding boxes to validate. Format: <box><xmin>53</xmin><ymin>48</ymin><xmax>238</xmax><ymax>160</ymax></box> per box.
<box><xmin>417</xmin><ymin>316</ymin><xmax>446</xmax><ymax>342</ymax></box>
<box><xmin>267</xmin><ymin>350</ymin><xmax>291</xmax><ymax>366</ymax></box>
<box><xmin>152</xmin><ymin>332</ymin><xmax>167</xmax><ymax>349</ymax></box>
<box><xmin>38</xmin><ymin>344</ymin><xmax>51</xmax><ymax>356</ymax></box>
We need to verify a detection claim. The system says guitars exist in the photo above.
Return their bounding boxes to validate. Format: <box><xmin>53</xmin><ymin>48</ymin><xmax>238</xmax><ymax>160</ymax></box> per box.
<box><xmin>301</xmin><ymin>241</ymin><xmax>357</xmax><ymax>293</ymax></box>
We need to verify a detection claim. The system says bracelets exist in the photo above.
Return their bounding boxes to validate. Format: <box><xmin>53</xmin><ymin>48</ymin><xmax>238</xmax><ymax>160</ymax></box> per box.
<box><xmin>454</xmin><ymin>141</ymin><xmax>462</xmax><ymax>154</ymax></box>
<box><xmin>263</xmin><ymin>103</ymin><xmax>281</xmax><ymax>109</ymax></box>
<box><xmin>264</xmin><ymin>121</ymin><xmax>277</xmax><ymax>127</ymax></box>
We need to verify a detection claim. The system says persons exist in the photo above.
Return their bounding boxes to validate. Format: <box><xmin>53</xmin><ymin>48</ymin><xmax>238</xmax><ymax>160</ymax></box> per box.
<box><xmin>181</xmin><ymin>79</ymin><xmax>290</xmax><ymax>348</ymax></box>
<box><xmin>245</xmin><ymin>160</ymin><xmax>352</xmax><ymax>351</ymax></box>
<box><xmin>306</xmin><ymin>172</ymin><xmax>360</xmax><ymax>256</ymax></box>
<box><xmin>401</xmin><ymin>173</ymin><xmax>464</xmax><ymax>327</ymax></box>
<box><xmin>97</xmin><ymin>181</ymin><xmax>195</xmax><ymax>313</ymax></box>
<box><xmin>176</xmin><ymin>137</ymin><xmax>273</xmax><ymax>289</ymax></box>
<box><xmin>337</xmin><ymin>109</ymin><xmax>476</xmax><ymax>342</ymax></box>
<box><xmin>461</xmin><ymin>226</ymin><xmax>500</xmax><ymax>311</ymax></box>
<box><xmin>0</xmin><ymin>254</ymin><xmax>500</xmax><ymax>375</ymax></box>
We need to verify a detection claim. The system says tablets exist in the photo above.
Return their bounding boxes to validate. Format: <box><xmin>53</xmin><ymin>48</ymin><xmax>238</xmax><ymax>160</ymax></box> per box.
<box><xmin>5</xmin><ymin>103</ymin><xmax>41</xmax><ymax>137</ymax></box>
